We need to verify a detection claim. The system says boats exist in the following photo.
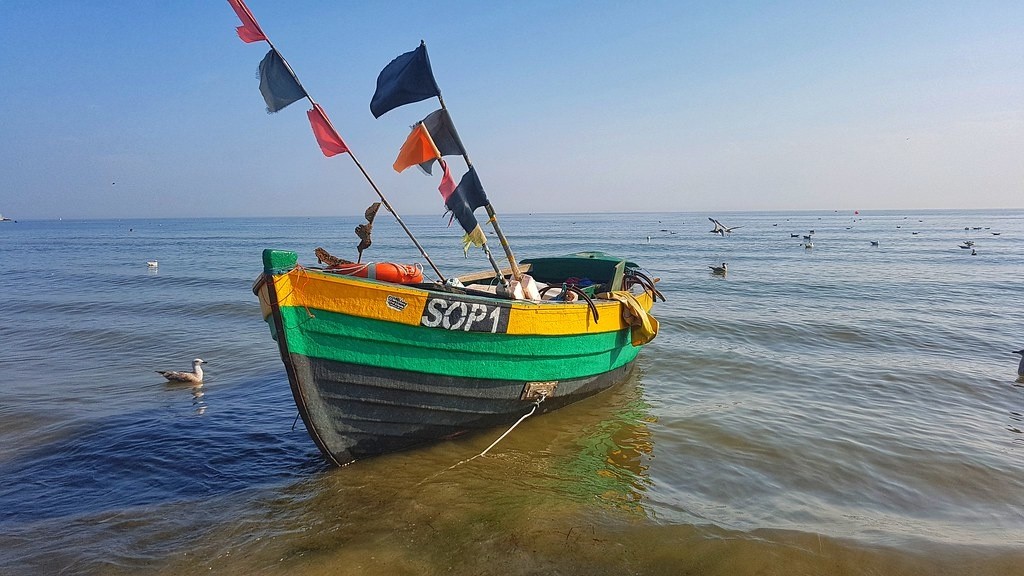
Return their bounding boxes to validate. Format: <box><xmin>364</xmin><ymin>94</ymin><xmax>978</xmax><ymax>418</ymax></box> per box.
<box><xmin>254</xmin><ymin>247</ymin><xmax>659</xmax><ymax>464</ymax></box>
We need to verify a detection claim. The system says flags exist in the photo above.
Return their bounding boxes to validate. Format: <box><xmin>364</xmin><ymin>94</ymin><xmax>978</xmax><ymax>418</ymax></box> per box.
<box><xmin>256</xmin><ymin>47</ymin><xmax>308</xmax><ymax>112</ymax></box>
<box><xmin>370</xmin><ymin>44</ymin><xmax>489</xmax><ymax>235</ymax></box>
<box><xmin>392</xmin><ymin>126</ymin><xmax>438</xmax><ymax>173</ymax></box>
<box><xmin>307</xmin><ymin>103</ymin><xmax>350</xmax><ymax>158</ymax></box>
<box><xmin>228</xmin><ymin>0</ymin><xmax>269</xmax><ymax>44</ymax></box>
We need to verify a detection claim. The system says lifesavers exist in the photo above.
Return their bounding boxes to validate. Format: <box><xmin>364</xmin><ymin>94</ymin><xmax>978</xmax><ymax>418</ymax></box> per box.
<box><xmin>325</xmin><ymin>261</ymin><xmax>421</xmax><ymax>284</ymax></box>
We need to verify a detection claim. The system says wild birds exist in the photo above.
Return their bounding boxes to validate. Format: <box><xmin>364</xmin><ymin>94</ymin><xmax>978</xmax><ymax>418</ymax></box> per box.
<box><xmin>708</xmin><ymin>263</ymin><xmax>728</xmax><ymax>274</ymax></box>
<box><xmin>896</xmin><ymin>217</ymin><xmax>923</xmax><ymax>235</ymax></box>
<box><xmin>708</xmin><ymin>217</ymin><xmax>745</xmax><ymax>237</ymax></box>
<box><xmin>790</xmin><ymin>230</ymin><xmax>815</xmax><ymax>250</ymax></box>
<box><xmin>773</xmin><ymin>224</ymin><xmax>777</xmax><ymax>226</ymax></box>
<box><xmin>146</xmin><ymin>260</ymin><xmax>159</xmax><ymax>268</ymax></box>
<box><xmin>846</xmin><ymin>216</ymin><xmax>862</xmax><ymax>229</ymax></box>
<box><xmin>787</xmin><ymin>219</ymin><xmax>789</xmax><ymax>221</ymax></box>
<box><xmin>1012</xmin><ymin>350</ymin><xmax>1024</xmax><ymax>376</ymax></box>
<box><xmin>870</xmin><ymin>241</ymin><xmax>879</xmax><ymax>246</ymax></box>
<box><xmin>647</xmin><ymin>220</ymin><xmax>685</xmax><ymax>240</ymax></box>
<box><xmin>154</xmin><ymin>358</ymin><xmax>208</xmax><ymax>384</ymax></box>
<box><xmin>957</xmin><ymin>226</ymin><xmax>1002</xmax><ymax>256</ymax></box>
